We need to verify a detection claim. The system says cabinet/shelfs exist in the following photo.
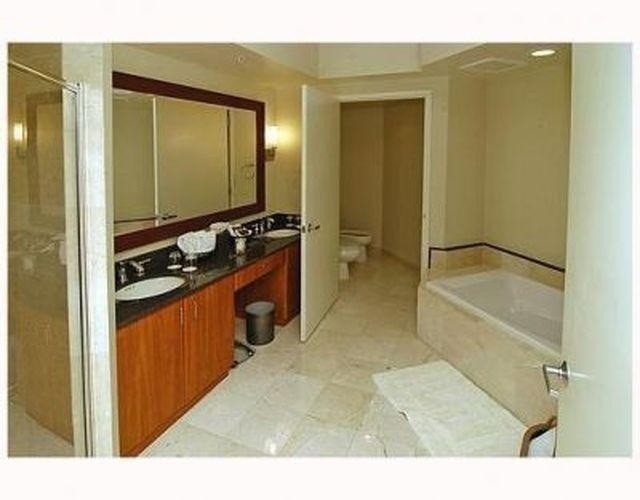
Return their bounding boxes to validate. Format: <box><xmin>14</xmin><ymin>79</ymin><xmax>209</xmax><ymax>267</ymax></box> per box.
<box><xmin>238</xmin><ymin>243</ymin><xmax>301</xmax><ymax>326</ymax></box>
<box><xmin>116</xmin><ymin>274</ymin><xmax>233</xmax><ymax>448</ymax></box>
<box><xmin>234</xmin><ymin>249</ymin><xmax>284</xmax><ymax>292</ymax></box>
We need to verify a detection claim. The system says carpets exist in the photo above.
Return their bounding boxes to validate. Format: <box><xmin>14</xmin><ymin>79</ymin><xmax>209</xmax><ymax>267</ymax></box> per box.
<box><xmin>375</xmin><ymin>359</ymin><xmax>530</xmax><ymax>458</ymax></box>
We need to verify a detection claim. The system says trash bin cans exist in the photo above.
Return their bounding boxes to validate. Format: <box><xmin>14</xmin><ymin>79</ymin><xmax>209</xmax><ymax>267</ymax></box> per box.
<box><xmin>245</xmin><ymin>302</ymin><xmax>274</xmax><ymax>345</ymax></box>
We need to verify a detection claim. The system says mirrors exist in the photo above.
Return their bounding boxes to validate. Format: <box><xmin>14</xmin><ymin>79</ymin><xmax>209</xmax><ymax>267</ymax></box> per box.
<box><xmin>112</xmin><ymin>69</ymin><xmax>269</xmax><ymax>255</ymax></box>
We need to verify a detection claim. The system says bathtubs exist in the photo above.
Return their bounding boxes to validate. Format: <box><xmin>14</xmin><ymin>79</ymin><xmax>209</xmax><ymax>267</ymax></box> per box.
<box><xmin>416</xmin><ymin>264</ymin><xmax>566</xmax><ymax>423</ymax></box>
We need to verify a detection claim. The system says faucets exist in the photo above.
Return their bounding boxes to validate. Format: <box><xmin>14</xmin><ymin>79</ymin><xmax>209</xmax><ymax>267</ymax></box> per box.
<box><xmin>125</xmin><ymin>260</ymin><xmax>144</xmax><ymax>278</ymax></box>
<box><xmin>259</xmin><ymin>219</ymin><xmax>266</xmax><ymax>233</ymax></box>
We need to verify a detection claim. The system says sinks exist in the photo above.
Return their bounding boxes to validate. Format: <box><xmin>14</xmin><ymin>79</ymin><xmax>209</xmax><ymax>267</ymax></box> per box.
<box><xmin>115</xmin><ymin>275</ymin><xmax>187</xmax><ymax>300</ymax></box>
<box><xmin>259</xmin><ymin>228</ymin><xmax>299</xmax><ymax>239</ymax></box>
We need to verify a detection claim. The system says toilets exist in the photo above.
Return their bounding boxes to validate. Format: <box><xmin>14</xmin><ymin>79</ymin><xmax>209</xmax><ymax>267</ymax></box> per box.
<box><xmin>339</xmin><ymin>230</ymin><xmax>372</xmax><ymax>263</ymax></box>
<box><xmin>339</xmin><ymin>236</ymin><xmax>360</xmax><ymax>279</ymax></box>
